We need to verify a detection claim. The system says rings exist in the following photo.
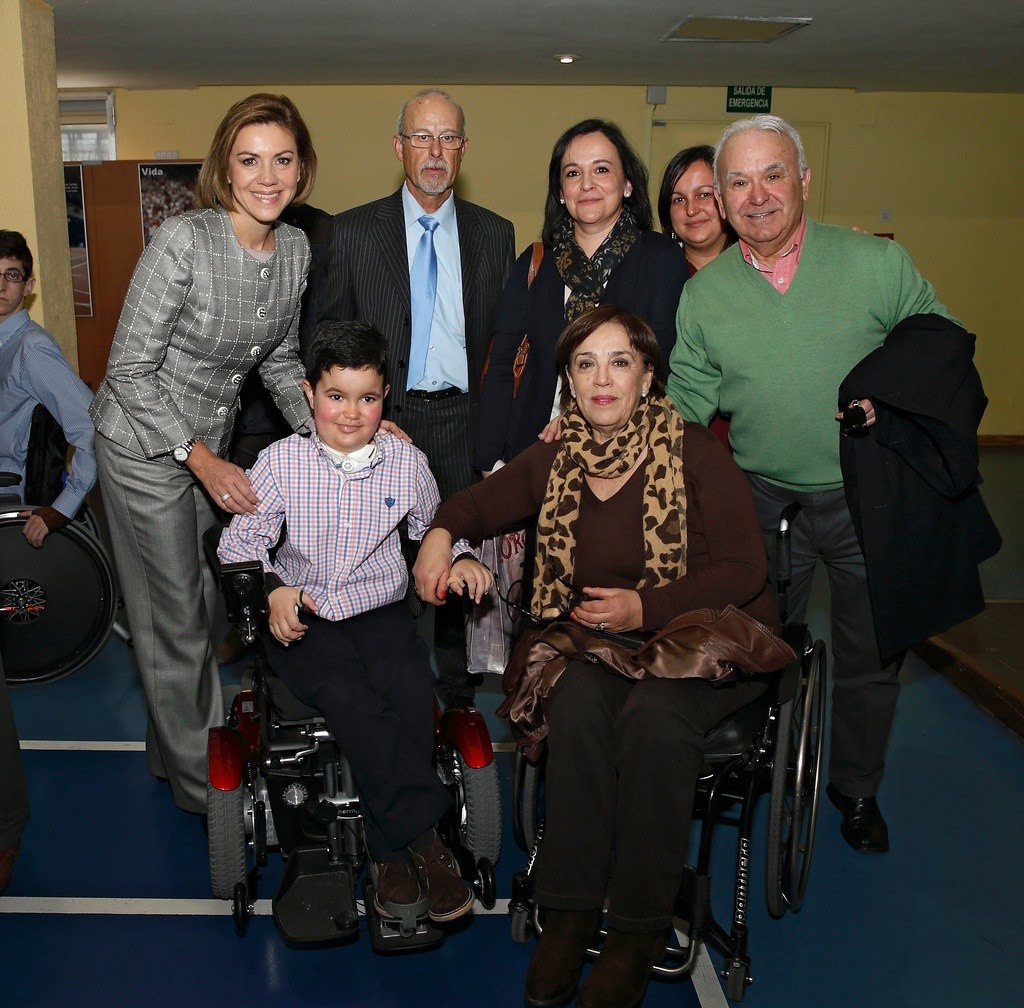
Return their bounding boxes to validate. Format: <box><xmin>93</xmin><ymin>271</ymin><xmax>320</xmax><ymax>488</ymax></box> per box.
<box><xmin>594</xmin><ymin>622</ymin><xmax>605</xmax><ymax>631</ymax></box>
<box><xmin>220</xmin><ymin>494</ymin><xmax>231</xmax><ymax>502</ymax></box>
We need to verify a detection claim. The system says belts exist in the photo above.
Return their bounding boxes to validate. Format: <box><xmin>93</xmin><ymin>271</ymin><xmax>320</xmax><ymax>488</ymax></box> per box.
<box><xmin>406</xmin><ymin>387</ymin><xmax>462</xmax><ymax>401</ymax></box>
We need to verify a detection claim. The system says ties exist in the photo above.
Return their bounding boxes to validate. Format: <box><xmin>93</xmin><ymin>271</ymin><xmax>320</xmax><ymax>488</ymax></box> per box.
<box><xmin>407</xmin><ymin>216</ymin><xmax>440</xmax><ymax>389</ymax></box>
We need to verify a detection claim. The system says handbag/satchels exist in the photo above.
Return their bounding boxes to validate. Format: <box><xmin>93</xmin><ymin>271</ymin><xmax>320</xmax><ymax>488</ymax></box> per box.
<box><xmin>479</xmin><ymin>242</ymin><xmax>544</xmax><ymax>402</ymax></box>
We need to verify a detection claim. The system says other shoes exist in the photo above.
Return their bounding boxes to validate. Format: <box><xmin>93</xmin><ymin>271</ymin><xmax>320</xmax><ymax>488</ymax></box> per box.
<box><xmin>435</xmin><ymin>677</ymin><xmax>475</xmax><ymax>709</ymax></box>
<box><xmin>578</xmin><ymin>927</ymin><xmax>673</xmax><ymax>1008</ymax></box>
<box><xmin>525</xmin><ymin>906</ymin><xmax>603</xmax><ymax>1008</ymax></box>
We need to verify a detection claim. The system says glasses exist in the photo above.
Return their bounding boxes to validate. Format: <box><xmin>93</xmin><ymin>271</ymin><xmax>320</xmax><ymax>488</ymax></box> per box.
<box><xmin>493</xmin><ymin>555</ymin><xmax>590</xmax><ymax>625</ymax></box>
<box><xmin>0</xmin><ymin>272</ymin><xmax>29</xmax><ymax>282</ymax></box>
<box><xmin>399</xmin><ymin>132</ymin><xmax>465</xmax><ymax>150</ymax></box>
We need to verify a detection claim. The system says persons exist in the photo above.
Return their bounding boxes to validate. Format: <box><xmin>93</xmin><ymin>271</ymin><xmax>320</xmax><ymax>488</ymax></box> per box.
<box><xmin>88</xmin><ymin>94</ymin><xmax>414</xmax><ymax>814</ymax></box>
<box><xmin>0</xmin><ymin>228</ymin><xmax>98</xmax><ymax>549</ymax></box>
<box><xmin>214</xmin><ymin>319</ymin><xmax>476</xmax><ymax>923</ymax></box>
<box><xmin>398</xmin><ymin>301</ymin><xmax>781</xmax><ymax>1008</ymax></box>
<box><xmin>232</xmin><ymin>88</ymin><xmax>871</xmax><ymax>708</ymax></box>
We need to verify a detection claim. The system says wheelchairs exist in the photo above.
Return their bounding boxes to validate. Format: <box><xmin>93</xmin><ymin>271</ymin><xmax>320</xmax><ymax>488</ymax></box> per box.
<box><xmin>202</xmin><ymin>525</ymin><xmax>499</xmax><ymax>946</ymax></box>
<box><xmin>0</xmin><ymin>402</ymin><xmax>119</xmax><ymax>687</ymax></box>
<box><xmin>511</xmin><ymin>493</ymin><xmax>832</xmax><ymax>1004</ymax></box>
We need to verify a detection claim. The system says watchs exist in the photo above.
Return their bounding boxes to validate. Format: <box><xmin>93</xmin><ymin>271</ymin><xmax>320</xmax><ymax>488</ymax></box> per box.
<box><xmin>172</xmin><ymin>432</ymin><xmax>201</xmax><ymax>463</ymax></box>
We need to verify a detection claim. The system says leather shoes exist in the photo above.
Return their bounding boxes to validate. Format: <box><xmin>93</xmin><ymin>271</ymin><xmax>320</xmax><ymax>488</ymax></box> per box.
<box><xmin>373</xmin><ymin>825</ymin><xmax>475</xmax><ymax>922</ymax></box>
<box><xmin>826</xmin><ymin>780</ymin><xmax>890</xmax><ymax>854</ymax></box>
